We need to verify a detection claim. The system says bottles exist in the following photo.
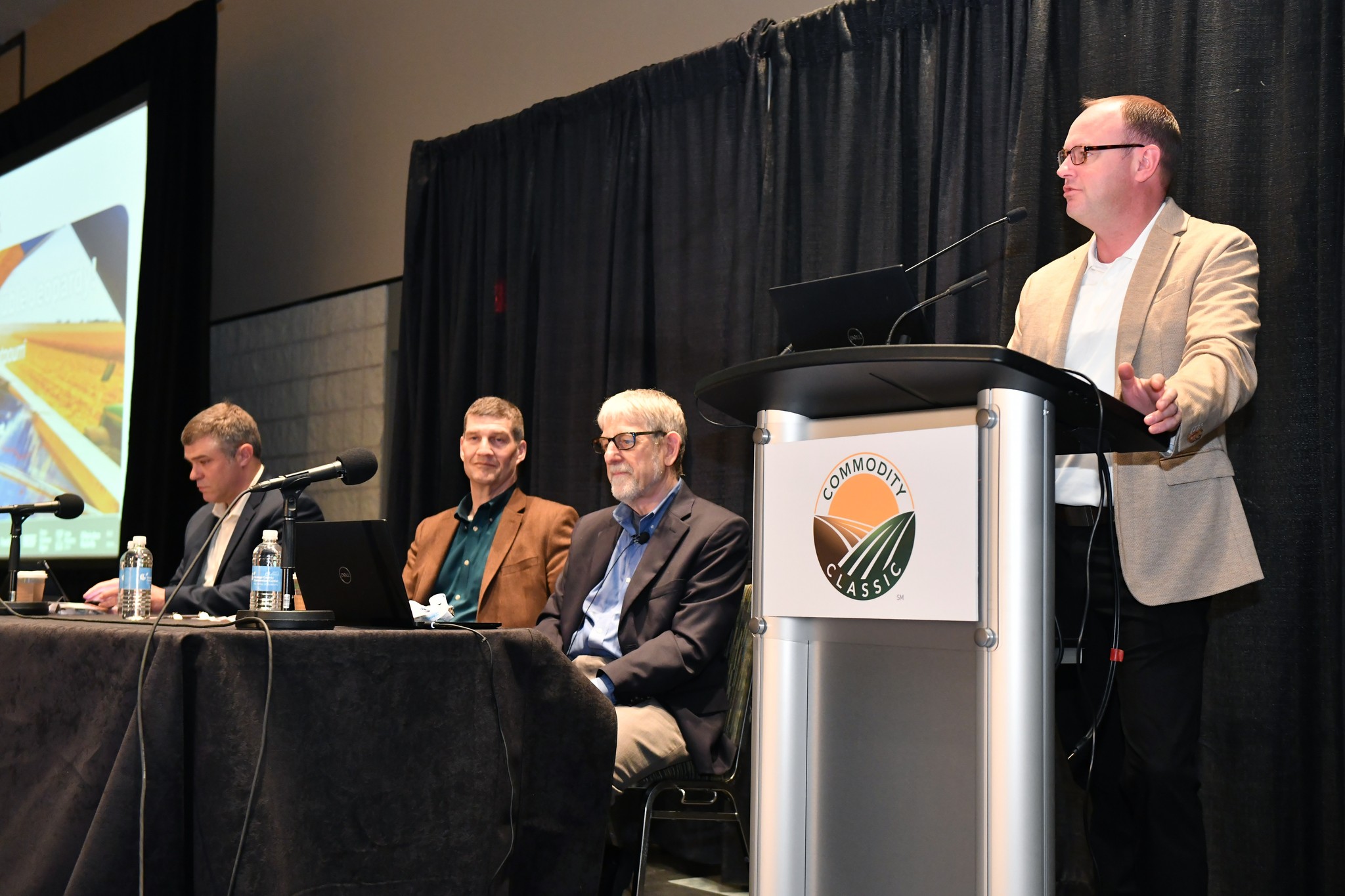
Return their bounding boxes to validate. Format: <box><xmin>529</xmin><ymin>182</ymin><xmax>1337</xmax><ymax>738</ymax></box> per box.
<box><xmin>118</xmin><ymin>541</ymin><xmax>135</xmax><ymax>617</ymax></box>
<box><xmin>122</xmin><ymin>536</ymin><xmax>153</xmax><ymax>621</ymax></box>
<box><xmin>249</xmin><ymin>530</ymin><xmax>282</xmax><ymax>611</ymax></box>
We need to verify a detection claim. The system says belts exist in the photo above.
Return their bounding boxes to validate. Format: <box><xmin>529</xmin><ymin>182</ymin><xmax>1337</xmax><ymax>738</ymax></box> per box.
<box><xmin>1056</xmin><ymin>505</ymin><xmax>1115</xmax><ymax>526</ymax></box>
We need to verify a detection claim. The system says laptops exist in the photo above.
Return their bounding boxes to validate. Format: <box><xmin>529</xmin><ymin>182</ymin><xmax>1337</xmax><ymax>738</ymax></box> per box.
<box><xmin>290</xmin><ymin>519</ymin><xmax>502</xmax><ymax>629</ymax></box>
<box><xmin>18</xmin><ymin>559</ymin><xmax>71</xmax><ymax>603</ymax></box>
<box><xmin>769</xmin><ymin>265</ymin><xmax>932</xmax><ymax>353</ymax></box>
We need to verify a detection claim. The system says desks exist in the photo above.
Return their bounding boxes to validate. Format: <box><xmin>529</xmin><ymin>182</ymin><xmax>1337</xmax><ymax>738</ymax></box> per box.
<box><xmin>0</xmin><ymin>601</ymin><xmax>620</xmax><ymax>896</ymax></box>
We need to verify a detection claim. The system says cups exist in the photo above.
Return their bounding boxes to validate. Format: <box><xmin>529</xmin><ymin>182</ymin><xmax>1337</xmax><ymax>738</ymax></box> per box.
<box><xmin>16</xmin><ymin>571</ymin><xmax>48</xmax><ymax>602</ymax></box>
<box><xmin>293</xmin><ymin>572</ymin><xmax>306</xmax><ymax>611</ymax></box>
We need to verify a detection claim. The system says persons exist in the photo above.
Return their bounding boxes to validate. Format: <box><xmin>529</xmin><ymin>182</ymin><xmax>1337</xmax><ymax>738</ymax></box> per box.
<box><xmin>85</xmin><ymin>401</ymin><xmax>325</xmax><ymax>619</ymax></box>
<box><xmin>401</xmin><ymin>398</ymin><xmax>584</xmax><ymax>629</ymax></box>
<box><xmin>1005</xmin><ymin>93</ymin><xmax>1264</xmax><ymax>896</ymax></box>
<box><xmin>527</xmin><ymin>387</ymin><xmax>750</xmax><ymax>807</ymax></box>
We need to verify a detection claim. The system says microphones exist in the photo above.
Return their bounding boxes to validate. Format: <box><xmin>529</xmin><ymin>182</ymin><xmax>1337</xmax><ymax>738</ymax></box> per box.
<box><xmin>905</xmin><ymin>208</ymin><xmax>1029</xmax><ymax>273</ymax></box>
<box><xmin>0</xmin><ymin>494</ymin><xmax>84</xmax><ymax>520</ymax></box>
<box><xmin>888</xmin><ymin>271</ymin><xmax>989</xmax><ymax>345</ymax></box>
<box><xmin>249</xmin><ymin>448</ymin><xmax>379</xmax><ymax>491</ymax></box>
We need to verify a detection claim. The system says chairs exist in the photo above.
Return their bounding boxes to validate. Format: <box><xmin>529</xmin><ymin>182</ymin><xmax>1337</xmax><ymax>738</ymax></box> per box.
<box><xmin>614</xmin><ymin>580</ymin><xmax>755</xmax><ymax>896</ymax></box>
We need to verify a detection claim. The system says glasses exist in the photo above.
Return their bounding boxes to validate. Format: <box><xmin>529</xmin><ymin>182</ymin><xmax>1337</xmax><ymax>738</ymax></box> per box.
<box><xmin>1058</xmin><ymin>144</ymin><xmax>1148</xmax><ymax>166</ymax></box>
<box><xmin>591</xmin><ymin>430</ymin><xmax>669</xmax><ymax>455</ymax></box>
